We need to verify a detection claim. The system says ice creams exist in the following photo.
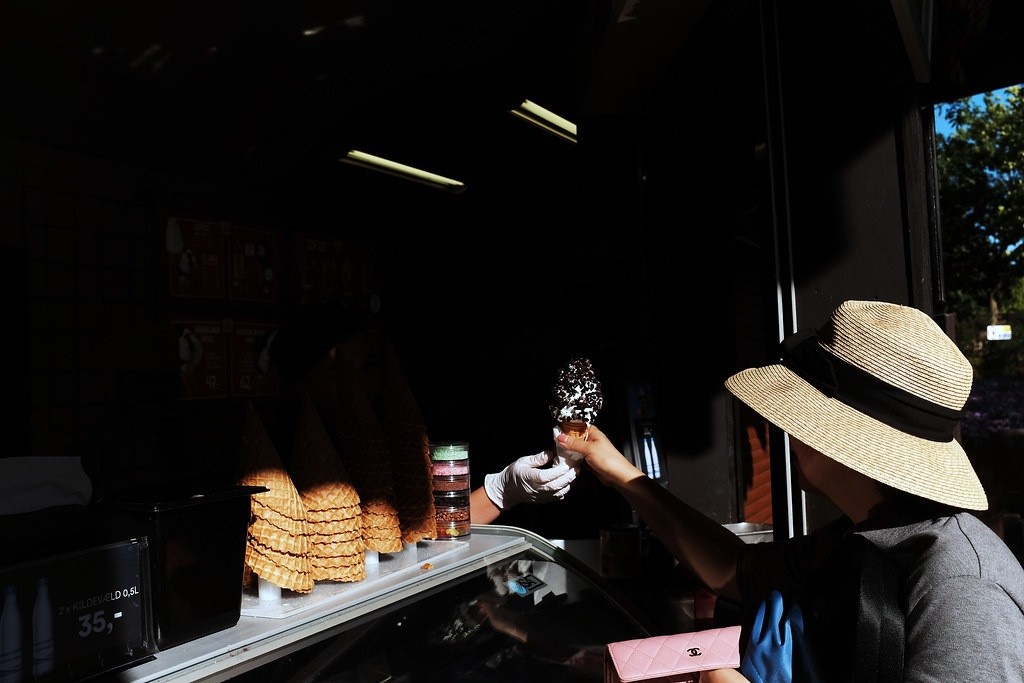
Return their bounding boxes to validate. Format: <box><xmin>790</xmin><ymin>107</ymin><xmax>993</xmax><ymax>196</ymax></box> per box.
<box><xmin>550</xmin><ymin>356</ymin><xmax>603</xmax><ymax>442</ymax></box>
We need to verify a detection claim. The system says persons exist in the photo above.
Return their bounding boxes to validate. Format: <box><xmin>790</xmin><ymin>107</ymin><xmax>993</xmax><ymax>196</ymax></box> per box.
<box><xmin>556</xmin><ymin>300</ymin><xmax>1024</xmax><ymax>683</ymax></box>
<box><xmin>264</xmin><ymin>313</ymin><xmax>580</xmax><ymax>682</ymax></box>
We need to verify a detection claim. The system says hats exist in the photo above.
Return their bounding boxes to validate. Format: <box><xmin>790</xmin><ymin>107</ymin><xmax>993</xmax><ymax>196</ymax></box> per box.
<box><xmin>725</xmin><ymin>300</ymin><xmax>988</xmax><ymax>512</ymax></box>
<box><xmin>270</xmin><ymin>303</ymin><xmax>382</xmax><ymax>384</ymax></box>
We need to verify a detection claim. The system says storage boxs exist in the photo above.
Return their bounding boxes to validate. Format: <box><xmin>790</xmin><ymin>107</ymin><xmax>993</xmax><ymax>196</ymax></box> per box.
<box><xmin>0</xmin><ymin>504</ymin><xmax>155</xmax><ymax>683</ymax></box>
<box><xmin>95</xmin><ymin>485</ymin><xmax>268</xmax><ymax>650</ymax></box>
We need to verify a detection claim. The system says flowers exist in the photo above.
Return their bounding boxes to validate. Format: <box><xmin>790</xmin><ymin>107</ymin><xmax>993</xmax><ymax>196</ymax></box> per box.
<box><xmin>960</xmin><ymin>379</ymin><xmax>1024</xmax><ymax>440</ymax></box>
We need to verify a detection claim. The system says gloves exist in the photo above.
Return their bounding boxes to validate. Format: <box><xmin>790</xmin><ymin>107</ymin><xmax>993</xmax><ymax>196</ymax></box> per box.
<box><xmin>483</xmin><ymin>451</ymin><xmax>582</xmax><ymax>512</ymax></box>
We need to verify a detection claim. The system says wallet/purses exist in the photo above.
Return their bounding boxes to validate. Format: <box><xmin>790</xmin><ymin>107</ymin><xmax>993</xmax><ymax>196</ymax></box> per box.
<box><xmin>603</xmin><ymin>624</ymin><xmax>751</xmax><ymax>683</ymax></box>
